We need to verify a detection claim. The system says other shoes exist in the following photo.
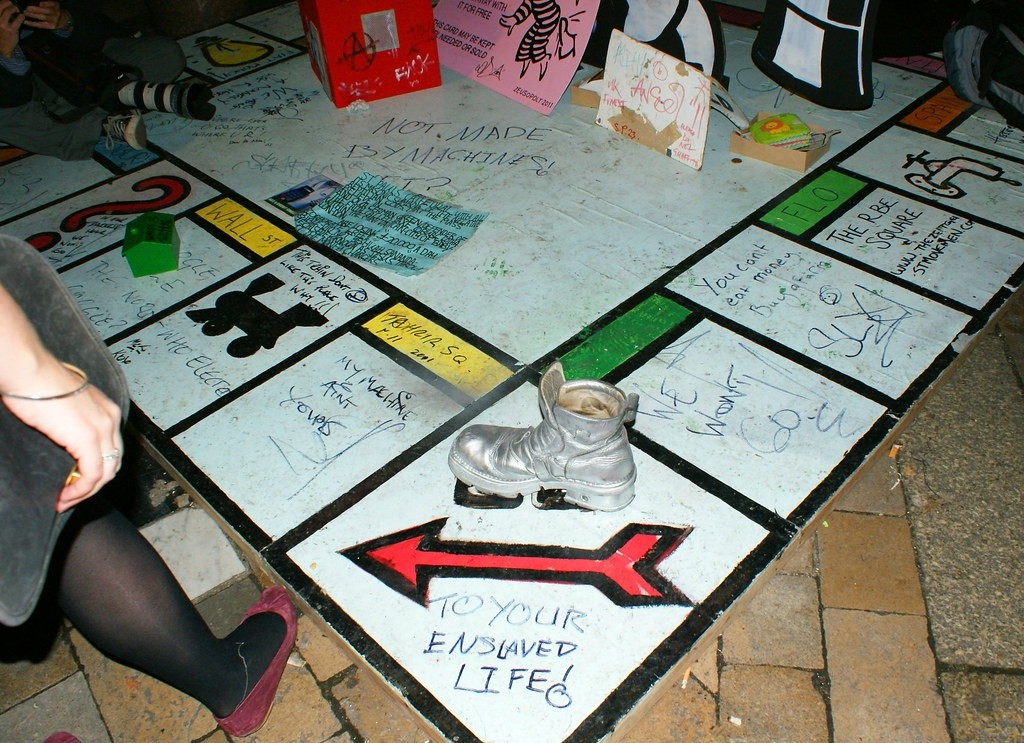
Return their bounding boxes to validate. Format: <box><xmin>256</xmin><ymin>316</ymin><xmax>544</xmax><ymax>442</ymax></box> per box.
<box><xmin>42</xmin><ymin>730</ymin><xmax>81</xmax><ymax>743</ymax></box>
<box><xmin>213</xmin><ymin>585</ymin><xmax>299</xmax><ymax>735</ymax></box>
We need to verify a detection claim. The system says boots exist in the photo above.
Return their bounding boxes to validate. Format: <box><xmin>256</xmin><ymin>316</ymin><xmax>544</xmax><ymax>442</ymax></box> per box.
<box><xmin>448</xmin><ymin>362</ymin><xmax>640</xmax><ymax>511</ymax></box>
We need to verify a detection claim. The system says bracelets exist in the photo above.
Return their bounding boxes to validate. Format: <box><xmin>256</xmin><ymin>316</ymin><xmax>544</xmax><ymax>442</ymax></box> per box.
<box><xmin>57</xmin><ymin>13</ymin><xmax>72</xmax><ymax>31</ymax></box>
<box><xmin>0</xmin><ymin>361</ymin><xmax>89</xmax><ymax>400</ymax></box>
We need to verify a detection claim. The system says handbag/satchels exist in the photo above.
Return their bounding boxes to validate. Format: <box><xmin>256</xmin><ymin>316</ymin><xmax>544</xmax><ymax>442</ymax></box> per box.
<box><xmin>18</xmin><ymin>19</ymin><xmax>134</xmax><ymax>124</ymax></box>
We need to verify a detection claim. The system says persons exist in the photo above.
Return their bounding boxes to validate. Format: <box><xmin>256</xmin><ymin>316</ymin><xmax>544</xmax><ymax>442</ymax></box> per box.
<box><xmin>0</xmin><ymin>290</ymin><xmax>296</xmax><ymax>743</ymax></box>
<box><xmin>0</xmin><ymin>0</ymin><xmax>187</xmax><ymax>163</ymax></box>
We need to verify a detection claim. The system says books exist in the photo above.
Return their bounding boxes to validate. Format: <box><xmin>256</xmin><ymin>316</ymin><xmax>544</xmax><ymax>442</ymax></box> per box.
<box><xmin>751</xmin><ymin>113</ymin><xmax>812</xmax><ymax>149</ymax></box>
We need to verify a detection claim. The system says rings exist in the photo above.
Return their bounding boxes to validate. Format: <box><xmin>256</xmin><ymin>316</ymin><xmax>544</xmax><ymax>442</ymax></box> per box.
<box><xmin>102</xmin><ymin>448</ymin><xmax>120</xmax><ymax>462</ymax></box>
<box><xmin>46</xmin><ymin>15</ymin><xmax>48</xmax><ymax>21</ymax></box>
